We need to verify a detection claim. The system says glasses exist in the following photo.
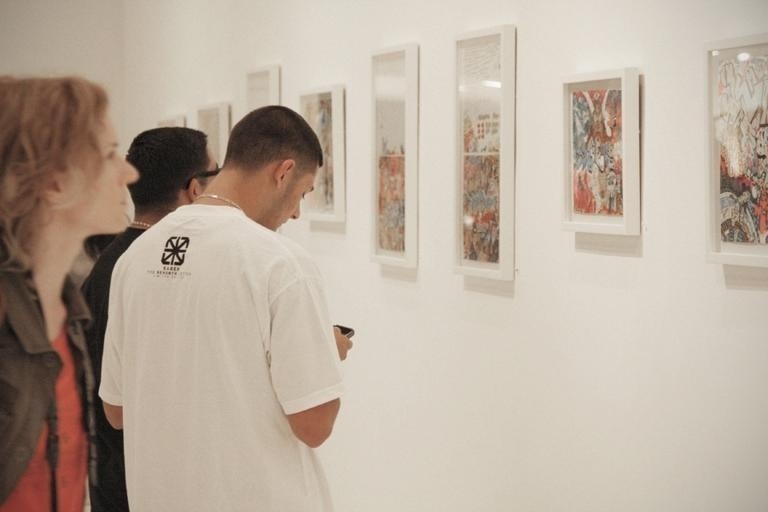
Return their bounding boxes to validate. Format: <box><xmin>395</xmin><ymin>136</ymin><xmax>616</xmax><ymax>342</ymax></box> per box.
<box><xmin>186</xmin><ymin>168</ymin><xmax>222</xmax><ymax>189</ymax></box>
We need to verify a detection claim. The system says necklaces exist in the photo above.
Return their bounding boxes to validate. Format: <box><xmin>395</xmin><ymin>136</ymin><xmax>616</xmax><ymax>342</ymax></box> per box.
<box><xmin>132</xmin><ymin>220</ymin><xmax>151</xmax><ymax>228</ymax></box>
<box><xmin>194</xmin><ymin>194</ymin><xmax>240</xmax><ymax>208</ymax></box>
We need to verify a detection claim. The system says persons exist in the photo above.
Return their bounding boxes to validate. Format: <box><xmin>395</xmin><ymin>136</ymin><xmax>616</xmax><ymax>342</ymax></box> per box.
<box><xmin>78</xmin><ymin>127</ymin><xmax>221</xmax><ymax>512</ymax></box>
<box><xmin>100</xmin><ymin>105</ymin><xmax>354</xmax><ymax>512</ymax></box>
<box><xmin>0</xmin><ymin>74</ymin><xmax>139</xmax><ymax>512</ymax></box>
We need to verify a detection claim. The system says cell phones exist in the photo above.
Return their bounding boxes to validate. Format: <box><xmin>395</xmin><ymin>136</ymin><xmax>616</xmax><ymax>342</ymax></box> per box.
<box><xmin>332</xmin><ymin>324</ymin><xmax>355</xmax><ymax>340</ymax></box>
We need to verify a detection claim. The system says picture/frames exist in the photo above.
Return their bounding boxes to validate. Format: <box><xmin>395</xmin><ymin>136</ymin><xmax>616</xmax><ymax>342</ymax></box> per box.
<box><xmin>560</xmin><ymin>67</ymin><xmax>641</xmax><ymax>236</ymax></box>
<box><xmin>300</xmin><ymin>83</ymin><xmax>345</xmax><ymax>224</ymax></box>
<box><xmin>704</xmin><ymin>32</ymin><xmax>768</xmax><ymax>268</ymax></box>
<box><xmin>367</xmin><ymin>24</ymin><xmax>517</xmax><ymax>283</ymax></box>
<box><xmin>195</xmin><ymin>64</ymin><xmax>281</xmax><ymax>170</ymax></box>
